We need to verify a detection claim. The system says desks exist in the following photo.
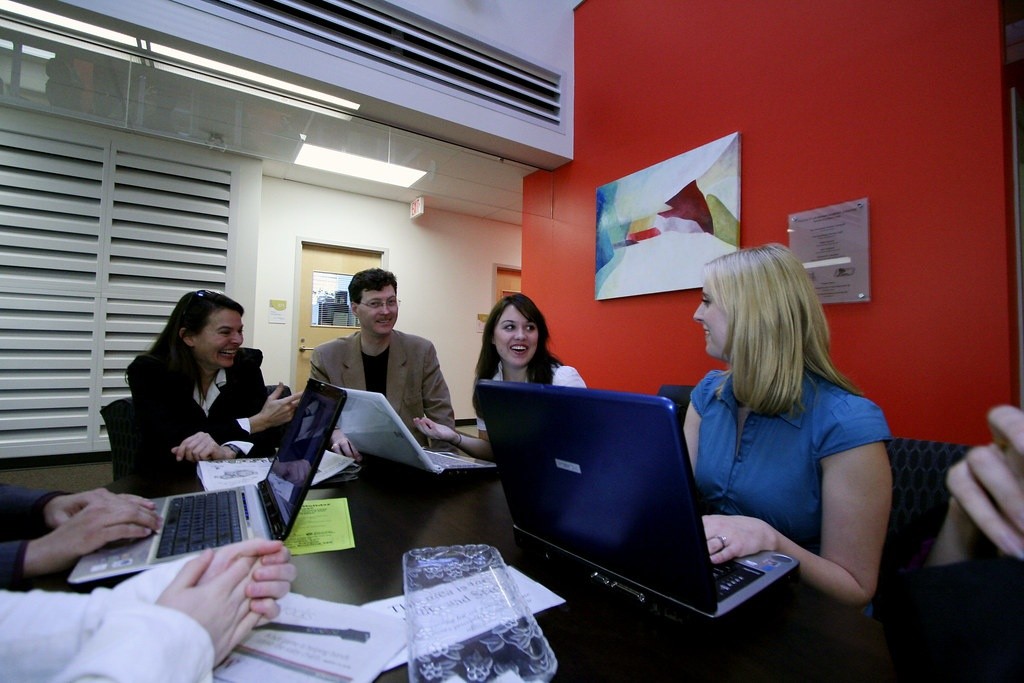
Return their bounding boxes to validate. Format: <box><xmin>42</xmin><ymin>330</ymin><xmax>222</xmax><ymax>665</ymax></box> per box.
<box><xmin>102</xmin><ymin>462</ymin><xmax>896</xmax><ymax>683</ymax></box>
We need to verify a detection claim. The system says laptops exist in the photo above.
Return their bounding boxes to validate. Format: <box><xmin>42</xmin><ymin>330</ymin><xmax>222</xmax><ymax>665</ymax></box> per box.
<box><xmin>475</xmin><ymin>380</ymin><xmax>799</xmax><ymax>622</ymax></box>
<box><xmin>68</xmin><ymin>377</ymin><xmax>347</xmax><ymax>594</ymax></box>
<box><xmin>338</xmin><ymin>387</ymin><xmax>498</xmax><ymax>474</ymax></box>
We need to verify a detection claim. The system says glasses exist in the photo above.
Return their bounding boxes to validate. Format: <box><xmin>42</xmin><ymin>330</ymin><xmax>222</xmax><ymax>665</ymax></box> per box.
<box><xmin>360</xmin><ymin>297</ymin><xmax>401</xmax><ymax>308</ymax></box>
<box><xmin>183</xmin><ymin>290</ymin><xmax>215</xmax><ymax>315</ymax></box>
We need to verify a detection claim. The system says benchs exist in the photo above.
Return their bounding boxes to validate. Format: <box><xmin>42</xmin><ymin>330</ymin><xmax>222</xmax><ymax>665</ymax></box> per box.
<box><xmin>659</xmin><ymin>385</ymin><xmax>971</xmax><ymax>592</ymax></box>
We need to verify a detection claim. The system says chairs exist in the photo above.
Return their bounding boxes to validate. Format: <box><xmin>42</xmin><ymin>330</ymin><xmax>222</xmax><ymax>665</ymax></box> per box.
<box><xmin>101</xmin><ymin>399</ymin><xmax>136</xmax><ymax>480</ymax></box>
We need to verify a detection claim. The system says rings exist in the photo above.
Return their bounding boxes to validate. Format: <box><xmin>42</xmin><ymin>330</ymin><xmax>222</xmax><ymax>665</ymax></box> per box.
<box><xmin>332</xmin><ymin>444</ymin><xmax>339</xmax><ymax>449</ymax></box>
<box><xmin>717</xmin><ymin>535</ymin><xmax>727</xmax><ymax>546</ymax></box>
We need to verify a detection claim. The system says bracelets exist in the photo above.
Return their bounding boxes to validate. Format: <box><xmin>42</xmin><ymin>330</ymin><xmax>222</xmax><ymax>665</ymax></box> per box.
<box><xmin>335</xmin><ymin>427</ymin><xmax>340</xmax><ymax>430</ymax></box>
<box><xmin>455</xmin><ymin>434</ymin><xmax>462</xmax><ymax>446</ymax></box>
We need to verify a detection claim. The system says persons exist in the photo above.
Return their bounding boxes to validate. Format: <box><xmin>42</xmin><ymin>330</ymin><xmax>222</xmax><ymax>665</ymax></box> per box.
<box><xmin>0</xmin><ymin>485</ymin><xmax>161</xmax><ymax>577</ymax></box>
<box><xmin>414</xmin><ymin>293</ymin><xmax>589</xmax><ymax>461</ymax></box>
<box><xmin>681</xmin><ymin>244</ymin><xmax>895</xmax><ymax>605</ymax></box>
<box><xmin>876</xmin><ymin>405</ymin><xmax>1024</xmax><ymax>683</ymax></box>
<box><xmin>309</xmin><ymin>269</ymin><xmax>458</xmax><ymax>462</ymax></box>
<box><xmin>1</xmin><ymin>537</ymin><xmax>299</xmax><ymax>681</ymax></box>
<box><xmin>126</xmin><ymin>290</ymin><xmax>304</xmax><ymax>475</ymax></box>
